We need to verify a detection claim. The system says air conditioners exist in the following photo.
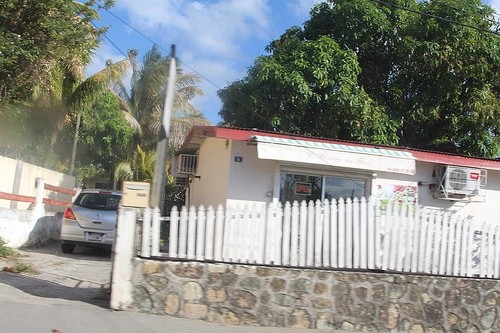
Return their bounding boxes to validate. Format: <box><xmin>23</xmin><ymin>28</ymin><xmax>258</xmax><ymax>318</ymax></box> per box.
<box><xmin>177</xmin><ymin>155</ymin><xmax>198</xmax><ymax>175</ymax></box>
<box><xmin>440</xmin><ymin>166</ymin><xmax>480</xmax><ymax>198</ymax></box>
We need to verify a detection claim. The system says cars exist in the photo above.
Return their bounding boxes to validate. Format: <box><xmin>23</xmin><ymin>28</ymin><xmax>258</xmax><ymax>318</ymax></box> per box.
<box><xmin>60</xmin><ymin>189</ymin><xmax>123</xmax><ymax>254</ymax></box>
